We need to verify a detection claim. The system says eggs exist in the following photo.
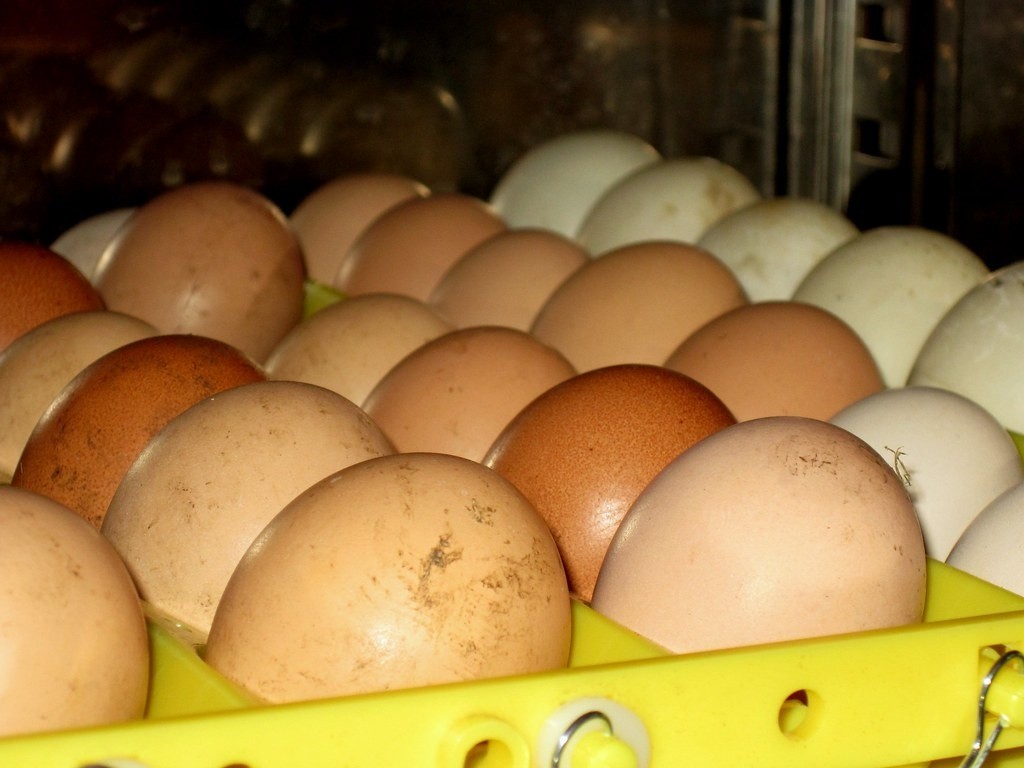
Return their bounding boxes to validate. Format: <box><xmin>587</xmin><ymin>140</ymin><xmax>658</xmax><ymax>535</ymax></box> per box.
<box><xmin>0</xmin><ymin>34</ymin><xmax>1024</xmax><ymax>737</ymax></box>
